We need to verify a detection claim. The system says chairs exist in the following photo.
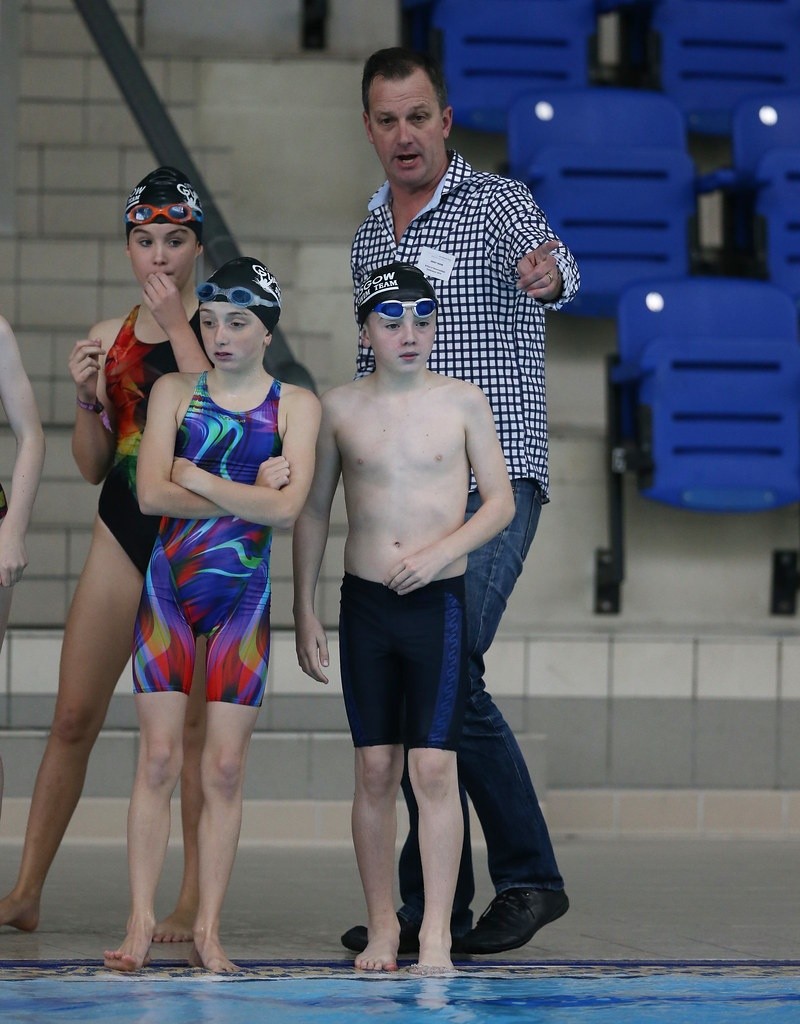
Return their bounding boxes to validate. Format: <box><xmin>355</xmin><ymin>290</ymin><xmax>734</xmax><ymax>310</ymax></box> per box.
<box><xmin>594</xmin><ymin>278</ymin><xmax>800</xmax><ymax>616</ymax></box>
<box><xmin>399</xmin><ymin>0</ymin><xmax>800</xmax><ymax>320</ymax></box>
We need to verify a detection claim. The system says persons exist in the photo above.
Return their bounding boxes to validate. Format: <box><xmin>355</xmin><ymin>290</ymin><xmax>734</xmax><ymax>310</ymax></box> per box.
<box><xmin>292</xmin><ymin>262</ymin><xmax>515</xmax><ymax>972</ymax></box>
<box><xmin>0</xmin><ymin>315</ymin><xmax>47</xmax><ymax>816</ymax></box>
<box><xmin>0</xmin><ymin>167</ymin><xmax>214</xmax><ymax>944</ymax></box>
<box><xmin>339</xmin><ymin>47</ymin><xmax>581</xmax><ymax>954</ymax></box>
<box><xmin>102</xmin><ymin>255</ymin><xmax>323</xmax><ymax>975</ymax></box>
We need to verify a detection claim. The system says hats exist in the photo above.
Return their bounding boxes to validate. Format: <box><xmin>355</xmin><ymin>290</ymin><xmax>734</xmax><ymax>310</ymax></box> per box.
<box><xmin>124</xmin><ymin>165</ymin><xmax>204</xmax><ymax>243</ymax></box>
<box><xmin>355</xmin><ymin>263</ymin><xmax>438</xmax><ymax>332</ymax></box>
<box><xmin>198</xmin><ymin>256</ymin><xmax>282</xmax><ymax>335</ymax></box>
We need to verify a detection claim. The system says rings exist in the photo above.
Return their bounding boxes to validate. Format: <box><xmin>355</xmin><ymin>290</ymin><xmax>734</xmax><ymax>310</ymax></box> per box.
<box><xmin>547</xmin><ymin>272</ymin><xmax>553</xmax><ymax>284</ymax></box>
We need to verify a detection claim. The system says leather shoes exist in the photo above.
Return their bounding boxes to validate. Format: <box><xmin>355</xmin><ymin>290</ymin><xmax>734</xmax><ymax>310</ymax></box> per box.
<box><xmin>460</xmin><ymin>885</ymin><xmax>570</xmax><ymax>956</ymax></box>
<box><xmin>340</xmin><ymin>913</ymin><xmax>459</xmax><ymax>954</ymax></box>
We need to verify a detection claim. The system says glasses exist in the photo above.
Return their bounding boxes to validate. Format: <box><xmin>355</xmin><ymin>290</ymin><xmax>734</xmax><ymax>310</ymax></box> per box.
<box><xmin>123</xmin><ymin>203</ymin><xmax>203</xmax><ymax>224</ymax></box>
<box><xmin>371</xmin><ymin>297</ymin><xmax>438</xmax><ymax>320</ymax></box>
<box><xmin>195</xmin><ymin>282</ymin><xmax>280</xmax><ymax>309</ymax></box>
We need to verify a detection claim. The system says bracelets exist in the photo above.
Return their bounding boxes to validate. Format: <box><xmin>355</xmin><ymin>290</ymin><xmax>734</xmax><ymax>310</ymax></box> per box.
<box><xmin>76</xmin><ymin>395</ymin><xmax>112</xmax><ymax>433</ymax></box>
